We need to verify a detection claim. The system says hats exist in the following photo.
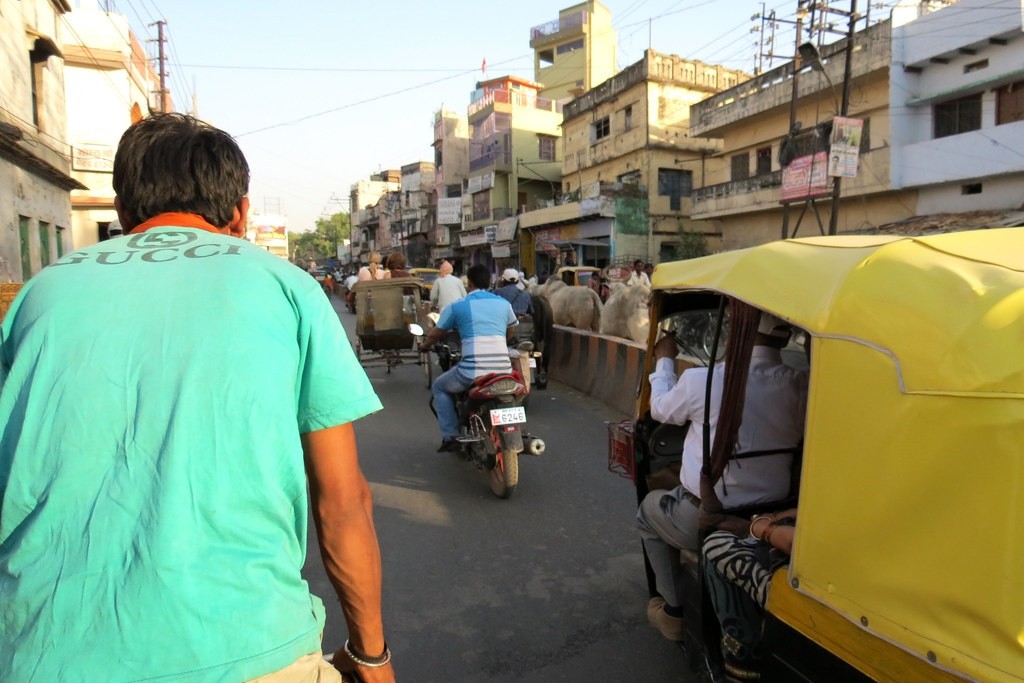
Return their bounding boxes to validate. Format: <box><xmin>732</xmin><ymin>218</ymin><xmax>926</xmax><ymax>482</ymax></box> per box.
<box><xmin>501</xmin><ymin>268</ymin><xmax>519</xmax><ymax>283</ymax></box>
<box><xmin>440</xmin><ymin>261</ymin><xmax>453</xmax><ymax>274</ymax></box>
<box><xmin>725</xmin><ymin>303</ymin><xmax>793</xmax><ymax>338</ymax></box>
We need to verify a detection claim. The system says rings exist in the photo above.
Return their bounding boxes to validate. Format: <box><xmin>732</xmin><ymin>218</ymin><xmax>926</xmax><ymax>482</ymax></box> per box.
<box><xmin>752</xmin><ymin>515</ymin><xmax>758</xmax><ymax>520</ymax></box>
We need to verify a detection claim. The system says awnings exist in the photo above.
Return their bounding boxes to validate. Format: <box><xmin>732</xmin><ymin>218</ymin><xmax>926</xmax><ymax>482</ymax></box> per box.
<box><xmin>547</xmin><ymin>240</ymin><xmax>610</xmax><ymax>247</ymax></box>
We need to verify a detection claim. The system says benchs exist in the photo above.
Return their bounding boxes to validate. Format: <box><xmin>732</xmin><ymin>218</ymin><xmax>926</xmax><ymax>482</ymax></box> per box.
<box><xmin>348</xmin><ymin>277</ymin><xmax>426</xmax><ymax>349</ymax></box>
<box><xmin>669</xmin><ymin>446</ymin><xmax>803</xmax><ymax>581</ymax></box>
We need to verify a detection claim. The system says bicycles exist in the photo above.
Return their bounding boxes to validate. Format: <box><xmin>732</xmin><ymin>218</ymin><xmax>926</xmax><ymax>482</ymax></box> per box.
<box><xmin>668</xmin><ymin>314</ymin><xmax>729</xmax><ymax>362</ymax></box>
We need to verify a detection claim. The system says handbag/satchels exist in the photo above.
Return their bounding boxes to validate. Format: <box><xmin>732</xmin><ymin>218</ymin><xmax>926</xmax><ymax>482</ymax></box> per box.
<box><xmin>767</xmin><ymin>516</ymin><xmax>796</xmax><ymax>574</ymax></box>
<box><xmin>507</xmin><ymin>313</ymin><xmax>536</xmax><ymax>346</ymax></box>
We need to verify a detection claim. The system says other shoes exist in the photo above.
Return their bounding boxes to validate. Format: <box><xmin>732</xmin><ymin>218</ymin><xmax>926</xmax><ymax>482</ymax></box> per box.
<box><xmin>647</xmin><ymin>597</ymin><xmax>684</xmax><ymax>641</ymax></box>
<box><xmin>396</xmin><ymin>360</ymin><xmax>403</xmax><ymax>364</ymax></box>
<box><xmin>721</xmin><ymin>638</ymin><xmax>761</xmax><ymax>679</ymax></box>
<box><xmin>437</xmin><ymin>439</ymin><xmax>463</xmax><ymax>452</ymax></box>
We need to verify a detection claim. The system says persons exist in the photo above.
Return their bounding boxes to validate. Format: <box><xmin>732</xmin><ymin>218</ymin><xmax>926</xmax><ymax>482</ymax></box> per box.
<box><xmin>421</xmin><ymin>263</ymin><xmax>519</xmax><ymax>453</ymax></box>
<box><xmin>0</xmin><ymin>112</ymin><xmax>396</xmax><ymax>683</ymax></box>
<box><xmin>637</xmin><ymin>312</ymin><xmax>811</xmax><ymax>679</ymax></box>
<box><xmin>291</xmin><ymin>251</ymin><xmax>654</xmax><ymax>325</ymax></box>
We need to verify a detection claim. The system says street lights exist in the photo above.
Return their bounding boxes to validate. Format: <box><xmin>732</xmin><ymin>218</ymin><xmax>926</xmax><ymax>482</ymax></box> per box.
<box><xmin>796</xmin><ymin>40</ymin><xmax>851</xmax><ymax>237</ymax></box>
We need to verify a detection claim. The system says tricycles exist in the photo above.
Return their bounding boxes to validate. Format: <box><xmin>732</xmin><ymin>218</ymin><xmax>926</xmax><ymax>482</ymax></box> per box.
<box><xmin>603</xmin><ymin>229</ymin><xmax>1024</xmax><ymax>682</ymax></box>
<box><xmin>346</xmin><ymin>277</ymin><xmax>433</xmax><ymax>391</ymax></box>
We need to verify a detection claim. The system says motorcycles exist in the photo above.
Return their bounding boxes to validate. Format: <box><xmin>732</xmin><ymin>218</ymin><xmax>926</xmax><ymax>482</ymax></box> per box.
<box><xmin>408</xmin><ymin>324</ymin><xmax>546</xmax><ymax>499</ymax></box>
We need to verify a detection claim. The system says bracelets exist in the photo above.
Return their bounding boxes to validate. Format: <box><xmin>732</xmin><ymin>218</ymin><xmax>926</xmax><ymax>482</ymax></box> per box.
<box><xmin>343</xmin><ymin>638</ymin><xmax>391</xmax><ymax>667</ymax></box>
<box><xmin>761</xmin><ymin>521</ymin><xmax>781</xmax><ymax>548</ymax></box>
<box><xmin>750</xmin><ymin>516</ymin><xmax>772</xmax><ymax>540</ymax></box>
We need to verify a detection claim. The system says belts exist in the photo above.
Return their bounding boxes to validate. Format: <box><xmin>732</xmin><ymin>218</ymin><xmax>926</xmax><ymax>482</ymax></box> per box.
<box><xmin>684</xmin><ymin>492</ymin><xmax>702</xmax><ymax>508</ymax></box>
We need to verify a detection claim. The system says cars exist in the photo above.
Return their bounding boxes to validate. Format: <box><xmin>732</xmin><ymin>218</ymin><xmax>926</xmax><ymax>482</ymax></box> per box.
<box><xmin>408</xmin><ymin>268</ymin><xmax>440</xmax><ymax>292</ymax></box>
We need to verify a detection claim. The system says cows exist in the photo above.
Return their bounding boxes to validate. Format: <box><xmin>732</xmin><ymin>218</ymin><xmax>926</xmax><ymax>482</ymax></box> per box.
<box><xmin>520</xmin><ymin>263</ymin><xmax>684</xmax><ymax>349</ymax></box>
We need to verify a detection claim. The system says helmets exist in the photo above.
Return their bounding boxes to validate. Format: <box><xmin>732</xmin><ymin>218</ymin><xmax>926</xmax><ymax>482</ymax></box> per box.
<box><xmin>107</xmin><ymin>219</ymin><xmax>123</xmax><ymax>239</ymax></box>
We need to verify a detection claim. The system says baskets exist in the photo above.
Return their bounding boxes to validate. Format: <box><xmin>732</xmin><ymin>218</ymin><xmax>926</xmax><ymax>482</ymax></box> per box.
<box><xmin>608</xmin><ymin>419</ymin><xmax>636</xmax><ymax>480</ymax></box>
